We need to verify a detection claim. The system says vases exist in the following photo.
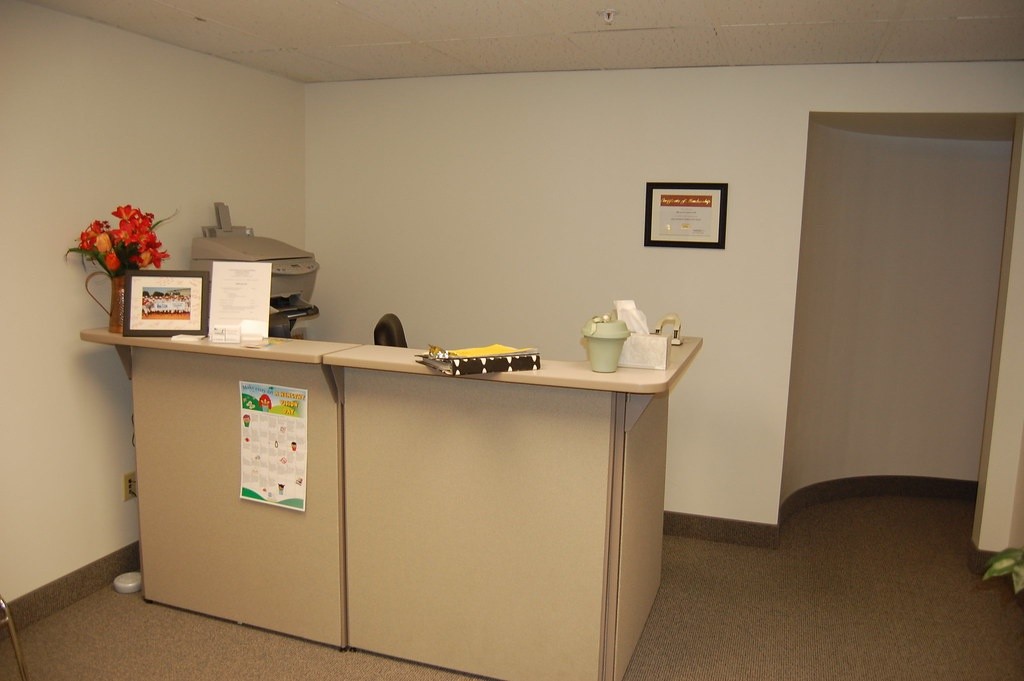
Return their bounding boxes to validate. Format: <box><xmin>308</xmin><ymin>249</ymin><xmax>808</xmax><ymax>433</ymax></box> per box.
<box><xmin>84</xmin><ymin>270</ymin><xmax>126</xmax><ymax>334</ymax></box>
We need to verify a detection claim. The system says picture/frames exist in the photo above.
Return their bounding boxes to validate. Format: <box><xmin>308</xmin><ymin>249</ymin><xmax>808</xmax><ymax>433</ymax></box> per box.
<box><xmin>123</xmin><ymin>269</ymin><xmax>211</xmax><ymax>339</ymax></box>
<box><xmin>644</xmin><ymin>182</ymin><xmax>728</xmax><ymax>249</ymax></box>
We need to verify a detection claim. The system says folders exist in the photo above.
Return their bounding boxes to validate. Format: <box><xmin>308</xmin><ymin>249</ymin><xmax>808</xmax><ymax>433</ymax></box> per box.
<box><xmin>415</xmin><ymin>352</ymin><xmax>540</xmax><ymax>377</ymax></box>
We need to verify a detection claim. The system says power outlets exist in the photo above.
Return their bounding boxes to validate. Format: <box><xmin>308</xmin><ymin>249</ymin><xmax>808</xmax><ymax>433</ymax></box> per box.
<box><xmin>124</xmin><ymin>470</ymin><xmax>138</xmax><ymax>501</ymax></box>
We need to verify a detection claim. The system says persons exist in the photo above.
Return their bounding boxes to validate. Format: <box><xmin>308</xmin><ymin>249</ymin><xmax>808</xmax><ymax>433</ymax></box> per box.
<box><xmin>142</xmin><ymin>287</ymin><xmax>190</xmax><ymax>320</ymax></box>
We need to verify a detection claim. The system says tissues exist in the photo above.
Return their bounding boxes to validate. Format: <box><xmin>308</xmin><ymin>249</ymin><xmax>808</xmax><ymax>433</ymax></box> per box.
<box><xmin>614</xmin><ymin>301</ymin><xmax>672</xmax><ymax>370</ymax></box>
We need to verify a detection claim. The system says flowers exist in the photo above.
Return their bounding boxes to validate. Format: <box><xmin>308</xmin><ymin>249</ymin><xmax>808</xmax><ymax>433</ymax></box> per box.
<box><xmin>56</xmin><ymin>203</ymin><xmax>180</xmax><ymax>276</ymax></box>
